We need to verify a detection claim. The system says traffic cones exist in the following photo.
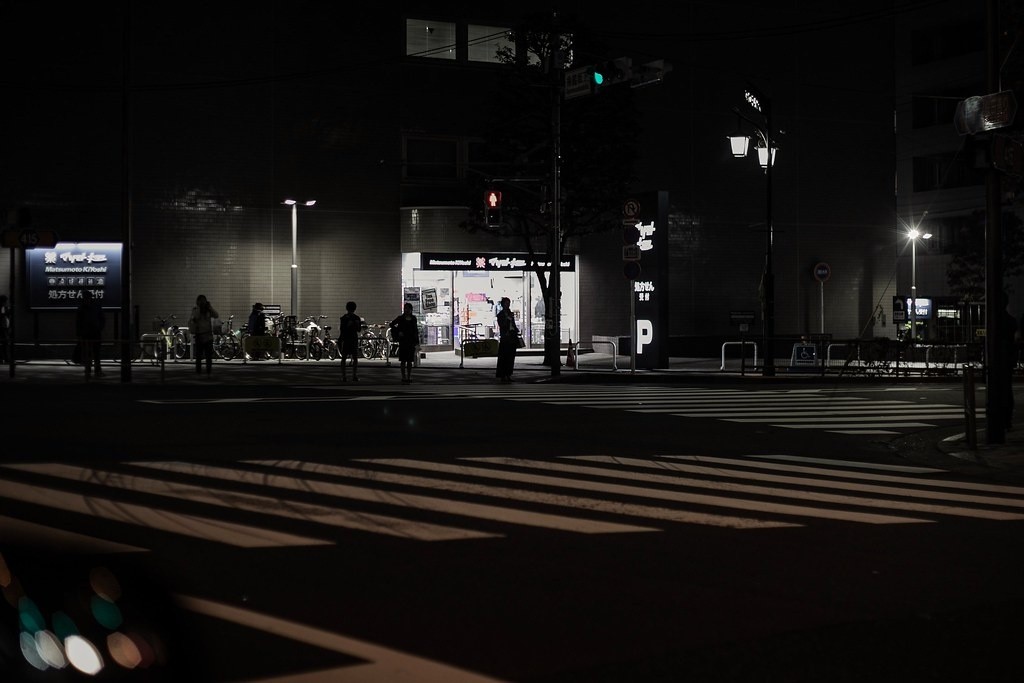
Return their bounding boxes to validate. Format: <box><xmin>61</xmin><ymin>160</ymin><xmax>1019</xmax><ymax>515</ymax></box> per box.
<box><xmin>566</xmin><ymin>339</ymin><xmax>576</xmax><ymax>367</ymax></box>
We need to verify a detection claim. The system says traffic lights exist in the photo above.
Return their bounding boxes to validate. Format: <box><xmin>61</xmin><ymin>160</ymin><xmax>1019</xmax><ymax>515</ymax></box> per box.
<box><xmin>586</xmin><ymin>60</ymin><xmax>620</xmax><ymax>90</ymax></box>
<box><xmin>484</xmin><ymin>191</ymin><xmax>503</xmax><ymax>228</ymax></box>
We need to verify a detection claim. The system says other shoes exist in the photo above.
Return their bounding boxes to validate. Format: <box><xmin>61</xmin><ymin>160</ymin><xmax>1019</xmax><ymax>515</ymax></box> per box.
<box><xmin>207</xmin><ymin>369</ymin><xmax>212</xmax><ymax>374</ymax></box>
<box><xmin>501</xmin><ymin>378</ymin><xmax>509</xmax><ymax>383</ymax></box>
<box><xmin>508</xmin><ymin>378</ymin><xmax>516</xmax><ymax>383</ymax></box>
<box><xmin>343</xmin><ymin>377</ymin><xmax>346</xmax><ymax>381</ymax></box>
<box><xmin>352</xmin><ymin>376</ymin><xmax>359</xmax><ymax>381</ymax></box>
<box><xmin>408</xmin><ymin>379</ymin><xmax>413</xmax><ymax>384</ymax></box>
<box><xmin>197</xmin><ymin>370</ymin><xmax>202</xmax><ymax>374</ymax></box>
<box><xmin>401</xmin><ymin>377</ymin><xmax>406</xmax><ymax>385</ymax></box>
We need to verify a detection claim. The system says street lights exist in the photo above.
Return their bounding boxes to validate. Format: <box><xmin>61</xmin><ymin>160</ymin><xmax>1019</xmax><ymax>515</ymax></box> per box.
<box><xmin>904</xmin><ymin>225</ymin><xmax>924</xmax><ymax>343</ymax></box>
<box><xmin>283</xmin><ymin>199</ymin><xmax>317</xmax><ymax>316</ymax></box>
<box><xmin>727</xmin><ymin>88</ymin><xmax>780</xmax><ymax>377</ymax></box>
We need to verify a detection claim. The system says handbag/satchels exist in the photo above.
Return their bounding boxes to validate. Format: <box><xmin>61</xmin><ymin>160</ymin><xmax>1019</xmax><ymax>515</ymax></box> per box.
<box><xmin>516</xmin><ymin>338</ymin><xmax>525</xmax><ymax>348</ymax></box>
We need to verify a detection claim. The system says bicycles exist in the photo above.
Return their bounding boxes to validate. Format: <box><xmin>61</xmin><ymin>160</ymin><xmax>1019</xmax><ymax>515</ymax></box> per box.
<box><xmin>152</xmin><ymin>313</ymin><xmax>398</xmax><ymax>361</ymax></box>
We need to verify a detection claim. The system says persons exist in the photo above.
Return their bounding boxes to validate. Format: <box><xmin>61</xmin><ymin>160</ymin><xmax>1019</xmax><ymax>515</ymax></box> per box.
<box><xmin>76</xmin><ymin>291</ymin><xmax>106</xmax><ymax>378</ymax></box>
<box><xmin>389</xmin><ymin>303</ymin><xmax>420</xmax><ymax>385</ymax></box>
<box><xmin>340</xmin><ymin>302</ymin><xmax>361</xmax><ymax>381</ymax></box>
<box><xmin>249</xmin><ymin>302</ymin><xmax>265</xmax><ymax>336</ymax></box>
<box><xmin>535</xmin><ymin>298</ymin><xmax>545</xmax><ymax>317</ymax></box>
<box><xmin>0</xmin><ymin>295</ymin><xmax>16</xmax><ymax>365</ymax></box>
<box><xmin>496</xmin><ymin>297</ymin><xmax>519</xmax><ymax>384</ymax></box>
<box><xmin>192</xmin><ymin>295</ymin><xmax>218</xmax><ymax>374</ymax></box>
